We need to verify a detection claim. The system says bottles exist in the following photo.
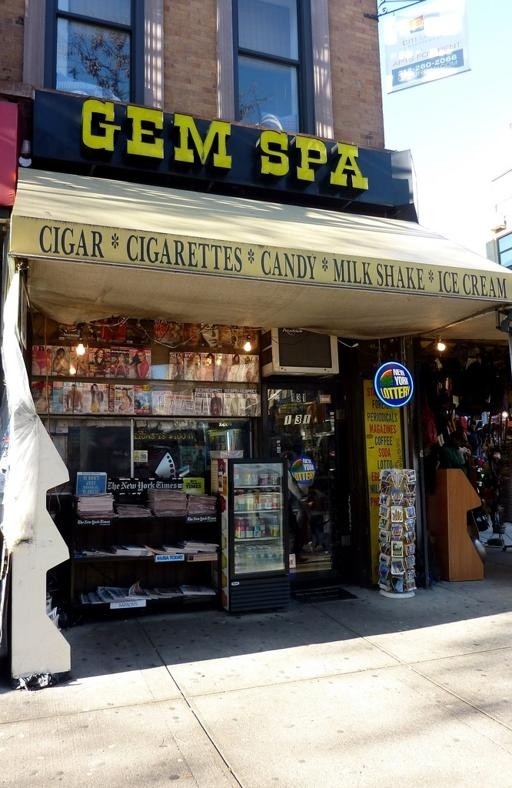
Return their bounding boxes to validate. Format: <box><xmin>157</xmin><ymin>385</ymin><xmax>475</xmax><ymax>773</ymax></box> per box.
<box><xmin>233</xmin><ymin>488</ymin><xmax>281</xmax><ymax>511</ymax></box>
<box><xmin>233</xmin><ymin>514</ymin><xmax>279</xmax><ymax>538</ymax></box>
<box><xmin>234</xmin><ymin>543</ymin><xmax>282</xmax><ymax>568</ymax></box>
<box><xmin>231</xmin><ymin>466</ymin><xmax>280</xmax><ymax>485</ymax></box>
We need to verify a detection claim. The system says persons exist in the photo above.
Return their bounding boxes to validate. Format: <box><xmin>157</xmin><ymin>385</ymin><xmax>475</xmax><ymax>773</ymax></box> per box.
<box><xmin>196</xmin><ymin>449</ymin><xmax>205</xmax><ymax>473</ymax></box>
<box><xmin>311</xmin><ymin>518</ymin><xmax>334</xmax><ymax>553</ymax></box>
<box><xmin>287</xmin><ymin>471</ymin><xmax>310</xmax><ymax>563</ymax></box>
<box><xmin>219</xmin><ymin>463</ymin><xmax>224</xmax><ymax>475</ymax></box>
<box><xmin>32</xmin><ymin>322</ymin><xmax>260</xmax><ymax>417</ymax></box>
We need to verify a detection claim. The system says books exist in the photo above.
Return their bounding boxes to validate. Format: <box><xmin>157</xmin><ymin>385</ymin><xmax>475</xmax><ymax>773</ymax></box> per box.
<box><xmin>70</xmin><ymin>487</ymin><xmax>220</xmax><ymax>605</ymax></box>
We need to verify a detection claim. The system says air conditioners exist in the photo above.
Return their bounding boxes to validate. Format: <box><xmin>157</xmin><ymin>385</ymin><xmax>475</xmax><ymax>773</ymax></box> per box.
<box><xmin>259</xmin><ymin>328</ymin><xmax>339</xmax><ymax>380</ymax></box>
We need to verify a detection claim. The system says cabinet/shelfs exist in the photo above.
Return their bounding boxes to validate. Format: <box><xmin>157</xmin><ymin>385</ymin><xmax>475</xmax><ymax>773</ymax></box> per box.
<box><xmin>424</xmin><ymin>468</ymin><xmax>485</xmax><ymax>582</ymax></box>
<box><xmin>66</xmin><ymin>492</ymin><xmax>222</xmax><ymax>627</ymax></box>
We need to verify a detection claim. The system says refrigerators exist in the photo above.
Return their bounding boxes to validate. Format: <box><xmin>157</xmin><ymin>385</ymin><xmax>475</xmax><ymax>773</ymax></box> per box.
<box><xmin>209</xmin><ymin>457</ymin><xmax>291</xmax><ymax>613</ymax></box>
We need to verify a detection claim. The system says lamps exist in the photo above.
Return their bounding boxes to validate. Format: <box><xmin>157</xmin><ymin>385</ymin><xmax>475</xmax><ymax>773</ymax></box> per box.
<box><xmin>68</xmin><ymin>350</ymin><xmax>76</xmax><ymax>376</ymax></box>
<box><xmin>243</xmin><ymin>333</ymin><xmax>252</xmax><ymax>353</ymax></box>
<box><xmin>76</xmin><ymin>327</ymin><xmax>85</xmax><ymax>356</ymax></box>
<box><xmin>434</xmin><ymin>335</ymin><xmax>446</xmax><ymax>352</ymax></box>
